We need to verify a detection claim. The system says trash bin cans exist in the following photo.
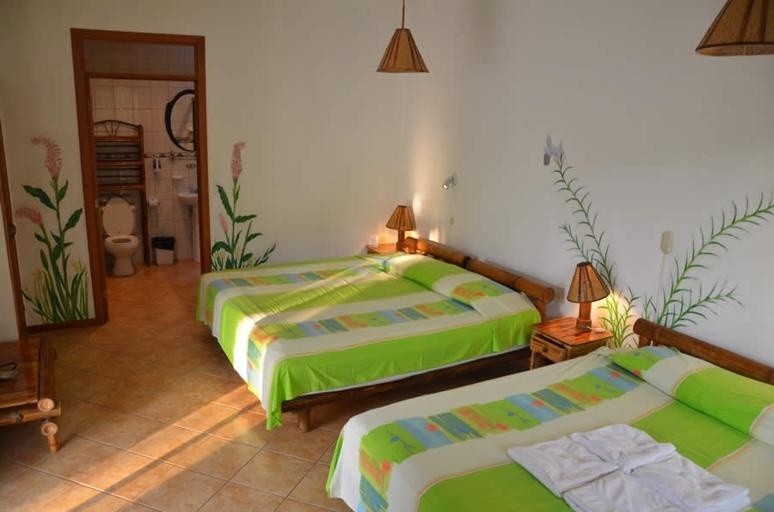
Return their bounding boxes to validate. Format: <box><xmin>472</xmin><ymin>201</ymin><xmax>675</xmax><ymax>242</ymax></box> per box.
<box><xmin>154</xmin><ymin>237</ymin><xmax>174</xmax><ymax>265</ymax></box>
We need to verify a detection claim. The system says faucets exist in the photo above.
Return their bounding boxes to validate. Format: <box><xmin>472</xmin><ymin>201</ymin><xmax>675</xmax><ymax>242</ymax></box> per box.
<box><xmin>191</xmin><ymin>189</ymin><xmax>198</xmax><ymax>194</ymax></box>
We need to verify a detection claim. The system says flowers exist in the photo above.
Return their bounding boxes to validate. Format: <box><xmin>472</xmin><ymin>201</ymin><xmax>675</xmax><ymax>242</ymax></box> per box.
<box><xmin>211</xmin><ymin>142</ymin><xmax>277</xmax><ymax>272</ymax></box>
<box><xmin>11</xmin><ymin>135</ymin><xmax>90</xmax><ymax>326</ymax></box>
<box><xmin>543</xmin><ymin>135</ymin><xmax>773</xmax><ymax>354</ymax></box>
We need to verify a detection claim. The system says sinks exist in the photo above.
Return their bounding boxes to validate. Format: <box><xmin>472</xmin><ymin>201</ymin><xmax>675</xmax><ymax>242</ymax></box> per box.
<box><xmin>178</xmin><ymin>192</ymin><xmax>199</xmax><ymax>208</ymax></box>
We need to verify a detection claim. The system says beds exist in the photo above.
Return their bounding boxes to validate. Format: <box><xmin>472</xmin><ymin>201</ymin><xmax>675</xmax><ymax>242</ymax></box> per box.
<box><xmin>340</xmin><ymin>318</ymin><xmax>773</xmax><ymax>512</ymax></box>
<box><xmin>198</xmin><ymin>238</ymin><xmax>556</xmax><ymax>432</ymax></box>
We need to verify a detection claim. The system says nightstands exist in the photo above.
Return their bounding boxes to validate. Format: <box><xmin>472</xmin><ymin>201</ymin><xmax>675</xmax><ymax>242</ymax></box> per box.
<box><xmin>530</xmin><ymin>316</ymin><xmax>614</xmax><ymax>370</ymax></box>
<box><xmin>366</xmin><ymin>243</ymin><xmax>397</xmax><ymax>254</ymax></box>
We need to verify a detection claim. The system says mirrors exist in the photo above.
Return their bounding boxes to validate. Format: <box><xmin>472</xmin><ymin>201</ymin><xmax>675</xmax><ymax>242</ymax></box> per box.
<box><xmin>164</xmin><ymin>90</ymin><xmax>198</xmax><ymax>152</ymax></box>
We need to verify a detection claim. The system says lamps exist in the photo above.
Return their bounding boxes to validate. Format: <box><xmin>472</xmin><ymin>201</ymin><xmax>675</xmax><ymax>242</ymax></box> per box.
<box><xmin>567</xmin><ymin>261</ymin><xmax>610</xmax><ymax>330</ymax></box>
<box><xmin>694</xmin><ymin>1</ymin><xmax>774</xmax><ymax>58</ymax></box>
<box><xmin>386</xmin><ymin>205</ymin><xmax>417</xmax><ymax>251</ymax></box>
<box><xmin>376</xmin><ymin>1</ymin><xmax>428</xmax><ymax>74</ymax></box>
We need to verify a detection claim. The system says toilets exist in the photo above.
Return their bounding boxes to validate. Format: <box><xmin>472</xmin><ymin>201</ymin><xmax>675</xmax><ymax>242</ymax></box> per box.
<box><xmin>100</xmin><ymin>196</ymin><xmax>140</xmax><ymax>277</ymax></box>
